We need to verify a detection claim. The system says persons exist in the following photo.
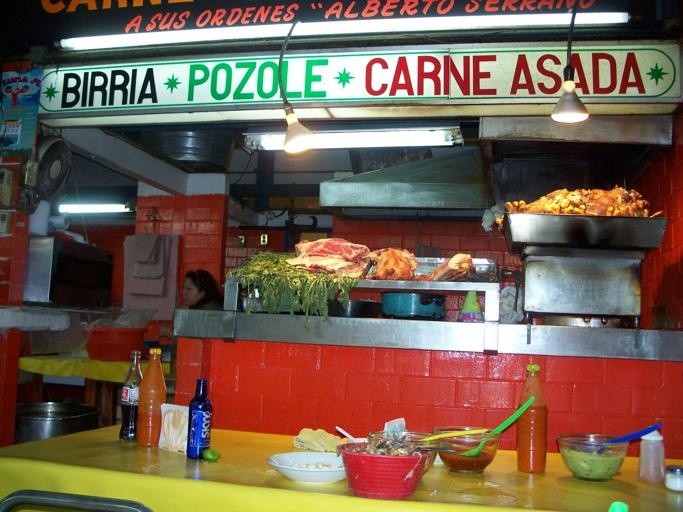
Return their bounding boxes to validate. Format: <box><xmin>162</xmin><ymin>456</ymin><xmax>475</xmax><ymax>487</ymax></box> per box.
<box><xmin>155</xmin><ymin>268</ymin><xmax>222</xmax><ymax>347</ymax></box>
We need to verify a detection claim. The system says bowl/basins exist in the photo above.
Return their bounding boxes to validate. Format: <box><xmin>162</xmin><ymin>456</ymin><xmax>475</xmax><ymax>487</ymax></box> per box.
<box><xmin>326</xmin><ymin>297</ymin><xmax>380</xmax><ymax>317</ymax></box>
<box><xmin>337</xmin><ymin>427</ymin><xmax>501</xmax><ymax>499</ymax></box>
<box><xmin>557</xmin><ymin>433</ymin><xmax>629</xmax><ymax>480</ymax></box>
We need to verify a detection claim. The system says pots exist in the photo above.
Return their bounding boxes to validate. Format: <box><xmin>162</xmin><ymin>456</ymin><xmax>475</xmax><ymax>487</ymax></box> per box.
<box><xmin>380</xmin><ymin>290</ymin><xmax>444</xmax><ymax>318</ymax></box>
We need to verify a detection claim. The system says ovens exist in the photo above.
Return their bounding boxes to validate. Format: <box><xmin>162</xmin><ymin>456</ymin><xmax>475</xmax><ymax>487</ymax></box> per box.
<box><xmin>23</xmin><ymin>236</ymin><xmax>115</xmax><ymax>309</ymax></box>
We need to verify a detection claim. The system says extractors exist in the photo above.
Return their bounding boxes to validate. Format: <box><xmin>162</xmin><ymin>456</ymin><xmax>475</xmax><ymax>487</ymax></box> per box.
<box><xmin>317</xmin><ymin>147</ymin><xmax>504</xmax><ymax>219</ymax></box>
<box><xmin>477</xmin><ymin>115</ymin><xmax>672</xmax><ymax>196</ymax></box>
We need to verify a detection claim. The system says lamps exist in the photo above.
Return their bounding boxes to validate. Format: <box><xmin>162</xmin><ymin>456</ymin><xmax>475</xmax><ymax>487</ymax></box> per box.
<box><xmin>550</xmin><ymin>0</ymin><xmax>590</xmax><ymax>124</ymax></box>
<box><xmin>242</xmin><ymin>124</ymin><xmax>468</xmax><ymax>153</ymax></box>
<box><xmin>54</xmin><ymin>10</ymin><xmax>637</xmax><ymax>53</ymax></box>
<box><xmin>60</xmin><ymin>200</ymin><xmax>132</xmax><ymax>215</ymax></box>
<box><xmin>276</xmin><ymin>1</ymin><xmax>314</xmax><ymax>154</ymax></box>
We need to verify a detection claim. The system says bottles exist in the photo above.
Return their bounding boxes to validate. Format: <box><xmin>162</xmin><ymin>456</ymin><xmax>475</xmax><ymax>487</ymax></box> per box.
<box><xmin>456</xmin><ymin>291</ymin><xmax>483</xmax><ymax>323</ymax></box>
<box><xmin>186</xmin><ymin>378</ymin><xmax>212</xmax><ymax>460</ymax></box>
<box><xmin>664</xmin><ymin>465</ymin><xmax>683</xmax><ymax>491</ymax></box>
<box><xmin>119</xmin><ymin>351</ymin><xmax>143</xmax><ymax>443</ymax></box>
<box><xmin>516</xmin><ymin>364</ymin><xmax>547</xmax><ymax>474</ymax></box>
<box><xmin>136</xmin><ymin>349</ymin><xmax>167</xmax><ymax>448</ymax></box>
<box><xmin>638</xmin><ymin>424</ymin><xmax>666</xmax><ymax>485</ymax></box>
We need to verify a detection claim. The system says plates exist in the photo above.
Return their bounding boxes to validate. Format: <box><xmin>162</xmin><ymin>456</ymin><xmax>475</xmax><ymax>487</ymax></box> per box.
<box><xmin>293</xmin><ymin>428</ymin><xmax>352</xmax><ymax>452</ymax></box>
<box><xmin>498</xmin><ymin>287</ymin><xmax>524</xmax><ymax>323</ymax></box>
<box><xmin>264</xmin><ymin>452</ymin><xmax>344</xmax><ymax>483</ymax></box>
